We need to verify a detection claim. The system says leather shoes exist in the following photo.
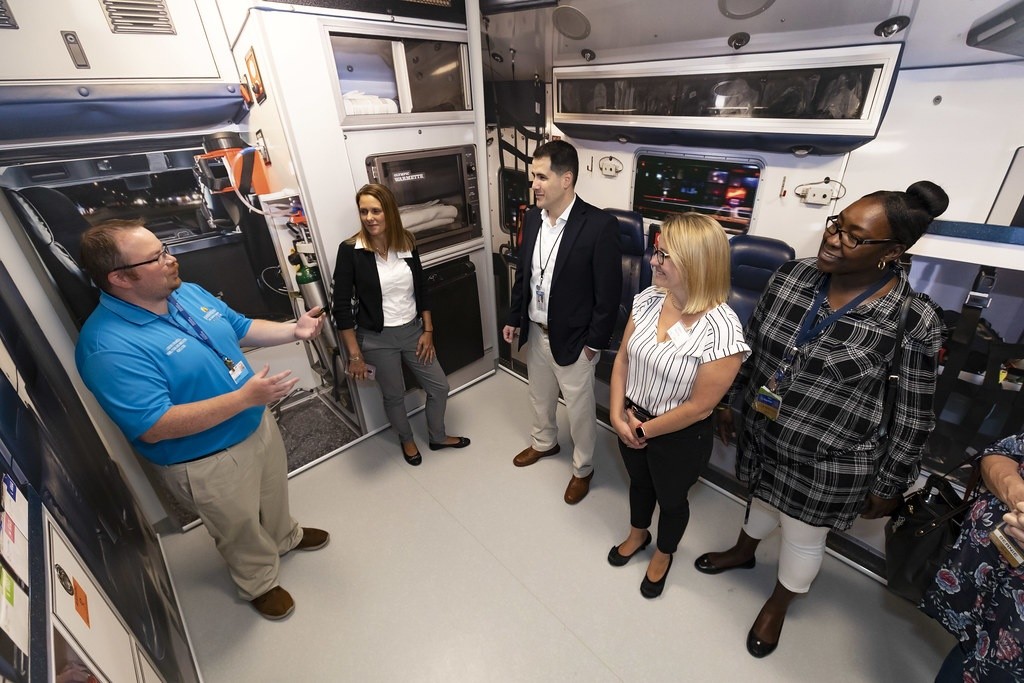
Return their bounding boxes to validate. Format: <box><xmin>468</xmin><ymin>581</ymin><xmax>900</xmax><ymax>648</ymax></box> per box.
<box><xmin>747</xmin><ymin>601</ymin><xmax>784</xmax><ymax>658</ymax></box>
<box><xmin>429</xmin><ymin>437</ymin><xmax>470</xmax><ymax>451</ymax></box>
<box><xmin>565</xmin><ymin>469</ymin><xmax>594</xmax><ymax>505</ymax></box>
<box><xmin>513</xmin><ymin>444</ymin><xmax>561</xmax><ymax>467</ymax></box>
<box><xmin>695</xmin><ymin>552</ymin><xmax>756</xmax><ymax>575</ymax></box>
<box><xmin>401</xmin><ymin>441</ymin><xmax>422</xmax><ymax>466</ymax></box>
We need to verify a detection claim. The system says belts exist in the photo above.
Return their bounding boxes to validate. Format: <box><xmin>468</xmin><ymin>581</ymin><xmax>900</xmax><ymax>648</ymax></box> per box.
<box><xmin>536</xmin><ymin>323</ymin><xmax>548</xmax><ymax>333</ymax></box>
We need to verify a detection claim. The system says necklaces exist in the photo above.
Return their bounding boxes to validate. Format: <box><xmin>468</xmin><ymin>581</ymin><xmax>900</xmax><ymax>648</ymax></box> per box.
<box><xmin>376</xmin><ymin>245</ymin><xmax>387</xmax><ymax>255</ymax></box>
<box><xmin>671</xmin><ymin>292</ymin><xmax>684</xmax><ymax>311</ymax></box>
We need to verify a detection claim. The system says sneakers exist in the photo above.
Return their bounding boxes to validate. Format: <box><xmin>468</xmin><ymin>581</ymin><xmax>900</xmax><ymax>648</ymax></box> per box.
<box><xmin>250</xmin><ymin>585</ymin><xmax>295</xmax><ymax>620</ymax></box>
<box><xmin>293</xmin><ymin>527</ymin><xmax>330</xmax><ymax>551</ymax></box>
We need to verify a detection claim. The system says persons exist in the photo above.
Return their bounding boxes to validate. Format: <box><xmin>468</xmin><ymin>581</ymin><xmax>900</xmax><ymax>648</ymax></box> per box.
<box><xmin>332</xmin><ymin>184</ymin><xmax>470</xmax><ymax>466</ymax></box>
<box><xmin>502</xmin><ymin>140</ymin><xmax>623</xmax><ymax>505</ymax></box>
<box><xmin>917</xmin><ymin>433</ymin><xmax>1024</xmax><ymax>683</ymax></box>
<box><xmin>74</xmin><ymin>217</ymin><xmax>329</xmax><ymax>621</ymax></box>
<box><xmin>607</xmin><ymin>212</ymin><xmax>752</xmax><ymax>599</ymax></box>
<box><xmin>693</xmin><ymin>181</ymin><xmax>950</xmax><ymax>659</ymax></box>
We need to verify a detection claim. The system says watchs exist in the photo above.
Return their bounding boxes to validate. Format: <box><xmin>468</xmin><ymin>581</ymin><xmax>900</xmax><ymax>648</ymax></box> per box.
<box><xmin>634</xmin><ymin>422</ymin><xmax>647</xmax><ymax>442</ymax></box>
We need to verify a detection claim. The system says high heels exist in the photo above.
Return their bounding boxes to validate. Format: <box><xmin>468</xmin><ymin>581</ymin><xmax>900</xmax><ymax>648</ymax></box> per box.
<box><xmin>608</xmin><ymin>530</ymin><xmax>652</xmax><ymax>567</ymax></box>
<box><xmin>640</xmin><ymin>553</ymin><xmax>673</xmax><ymax>598</ymax></box>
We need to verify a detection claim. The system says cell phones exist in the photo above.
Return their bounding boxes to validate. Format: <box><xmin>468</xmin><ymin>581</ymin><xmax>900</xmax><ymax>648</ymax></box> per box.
<box><xmin>344</xmin><ymin>361</ymin><xmax>376</xmax><ymax>381</ymax></box>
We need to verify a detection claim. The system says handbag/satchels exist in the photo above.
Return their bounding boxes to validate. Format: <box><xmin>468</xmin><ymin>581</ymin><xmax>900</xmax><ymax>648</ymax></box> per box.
<box><xmin>884</xmin><ymin>472</ymin><xmax>972</xmax><ymax>602</ymax></box>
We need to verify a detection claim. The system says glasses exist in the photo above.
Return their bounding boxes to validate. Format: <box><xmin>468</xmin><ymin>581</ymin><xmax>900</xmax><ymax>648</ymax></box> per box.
<box><xmin>825</xmin><ymin>215</ymin><xmax>899</xmax><ymax>249</ymax></box>
<box><xmin>653</xmin><ymin>244</ymin><xmax>670</xmax><ymax>265</ymax></box>
<box><xmin>110</xmin><ymin>243</ymin><xmax>169</xmax><ymax>272</ymax></box>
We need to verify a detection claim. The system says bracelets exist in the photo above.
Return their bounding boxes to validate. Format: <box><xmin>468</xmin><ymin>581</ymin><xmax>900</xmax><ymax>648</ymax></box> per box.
<box><xmin>424</xmin><ymin>330</ymin><xmax>432</xmax><ymax>332</ymax></box>
<box><xmin>349</xmin><ymin>351</ymin><xmax>363</xmax><ymax>362</ymax></box>
